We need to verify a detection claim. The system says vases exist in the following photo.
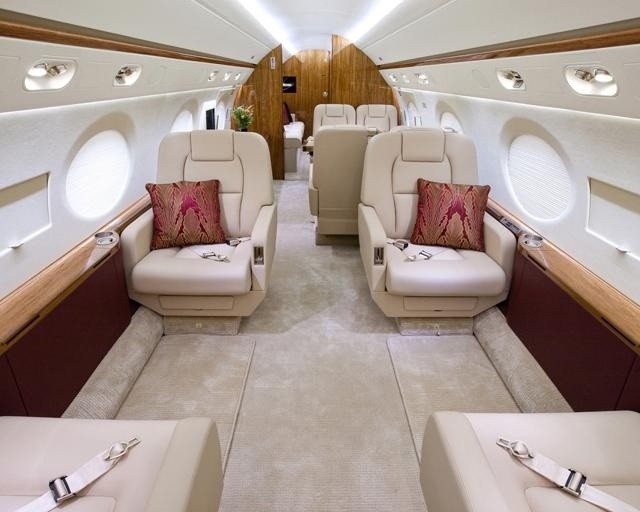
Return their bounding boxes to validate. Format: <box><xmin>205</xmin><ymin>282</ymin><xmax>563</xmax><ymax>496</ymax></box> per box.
<box><xmin>240</xmin><ymin>128</ymin><xmax>247</xmax><ymax>132</ymax></box>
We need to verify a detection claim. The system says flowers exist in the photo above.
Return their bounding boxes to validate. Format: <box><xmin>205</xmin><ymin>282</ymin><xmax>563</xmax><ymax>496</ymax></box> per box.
<box><xmin>232</xmin><ymin>106</ymin><xmax>254</xmax><ymax>130</ymax></box>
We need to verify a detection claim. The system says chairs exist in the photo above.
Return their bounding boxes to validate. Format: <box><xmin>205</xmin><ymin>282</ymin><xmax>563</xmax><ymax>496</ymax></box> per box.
<box><xmin>121</xmin><ymin>130</ymin><xmax>278</xmax><ymax>318</ymax></box>
<box><xmin>0</xmin><ymin>415</ymin><xmax>221</xmax><ymax>512</ymax></box>
<box><xmin>419</xmin><ymin>412</ymin><xmax>639</xmax><ymax>512</ymax></box>
<box><xmin>311</xmin><ymin>105</ymin><xmax>401</xmax><ymax>247</ymax></box>
<box><xmin>357</xmin><ymin>129</ymin><xmax>518</xmax><ymax>333</ymax></box>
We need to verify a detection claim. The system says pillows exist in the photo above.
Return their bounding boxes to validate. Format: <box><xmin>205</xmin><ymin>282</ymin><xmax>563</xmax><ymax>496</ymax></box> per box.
<box><xmin>410</xmin><ymin>177</ymin><xmax>492</xmax><ymax>250</ymax></box>
<box><xmin>146</xmin><ymin>180</ymin><xmax>226</xmax><ymax>249</ymax></box>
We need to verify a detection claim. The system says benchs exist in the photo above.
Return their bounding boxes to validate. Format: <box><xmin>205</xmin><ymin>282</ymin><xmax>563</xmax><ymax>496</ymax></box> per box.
<box><xmin>283</xmin><ymin>121</ymin><xmax>304</xmax><ymax>172</ymax></box>
